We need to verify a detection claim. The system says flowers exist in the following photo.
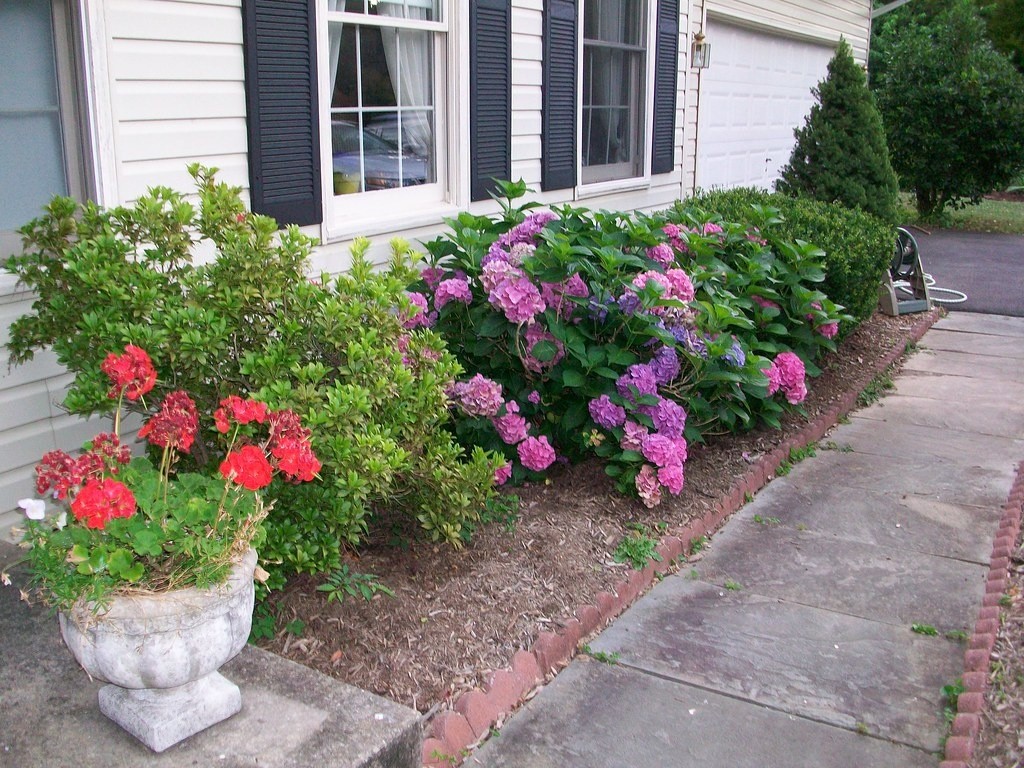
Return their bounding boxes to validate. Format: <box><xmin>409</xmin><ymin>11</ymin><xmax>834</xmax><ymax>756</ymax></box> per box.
<box><xmin>0</xmin><ymin>344</ymin><xmax>322</xmax><ymax>635</ymax></box>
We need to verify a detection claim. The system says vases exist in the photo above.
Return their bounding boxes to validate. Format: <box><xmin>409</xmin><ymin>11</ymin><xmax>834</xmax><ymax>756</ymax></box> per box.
<box><xmin>48</xmin><ymin>545</ymin><xmax>259</xmax><ymax>754</ymax></box>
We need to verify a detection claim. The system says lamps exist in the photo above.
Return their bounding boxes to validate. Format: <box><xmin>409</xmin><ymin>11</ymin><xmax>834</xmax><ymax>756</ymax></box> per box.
<box><xmin>691</xmin><ymin>29</ymin><xmax>711</xmax><ymax>68</ymax></box>
